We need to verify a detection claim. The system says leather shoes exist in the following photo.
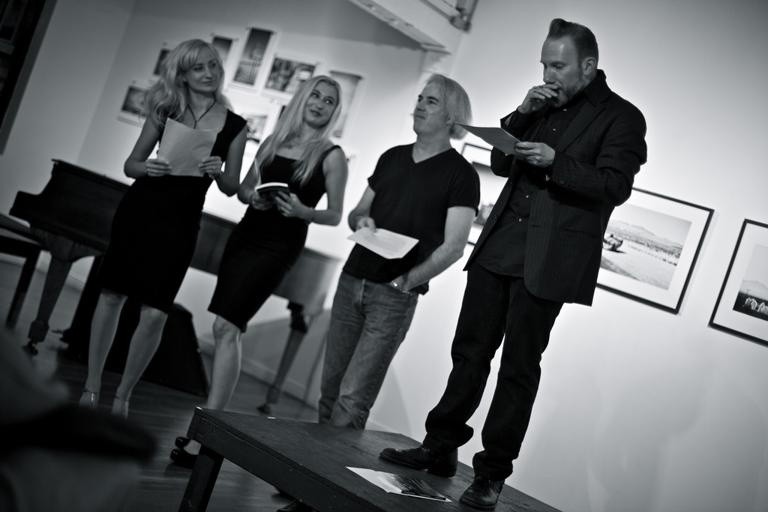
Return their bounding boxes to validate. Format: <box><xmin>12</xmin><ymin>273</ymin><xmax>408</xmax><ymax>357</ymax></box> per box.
<box><xmin>274</xmin><ymin>498</ymin><xmax>318</xmax><ymax>512</ymax></box>
<box><xmin>173</xmin><ymin>435</ymin><xmax>194</xmax><ymax>449</ymax></box>
<box><xmin>458</xmin><ymin>469</ymin><xmax>510</xmax><ymax>511</ymax></box>
<box><xmin>377</xmin><ymin>439</ymin><xmax>460</xmax><ymax>480</ymax></box>
<box><xmin>169</xmin><ymin>447</ymin><xmax>200</xmax><ymax>469</ymax></box>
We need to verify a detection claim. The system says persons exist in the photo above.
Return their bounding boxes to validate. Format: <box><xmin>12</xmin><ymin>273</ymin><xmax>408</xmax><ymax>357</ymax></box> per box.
<box><xmin>78</xmin><ymin>40</ymin><xmax>248</xmax><ymax>419</ymax></box>
<box><xmin>380</xmin><ymin>18</ymin><xmax>647</xmax><ymax>512</ymax></box>
<box><xmin>318</xmin><ymin>73</ymin><xmax>480</xmax><ymax>429</ymax></box>
<box><xmin>171</xmin><ymin>75</ymin><xmax>349</xmax><ymax>466</ymax></box>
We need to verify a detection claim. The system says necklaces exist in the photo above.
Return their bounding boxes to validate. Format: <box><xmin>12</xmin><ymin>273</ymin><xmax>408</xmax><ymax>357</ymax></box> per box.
<box><xmin>287</xmin><ymin>139</ymin><xmax>300</xmax><ymax>150</ymax></box>
<box><xmin>188</xmin><ymin>100</ymin><xmax>216</xmax><ymax>130</ymax></box>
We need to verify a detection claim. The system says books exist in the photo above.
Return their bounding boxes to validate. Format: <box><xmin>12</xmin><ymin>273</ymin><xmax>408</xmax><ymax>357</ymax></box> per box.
<box><xmin>255</xmin><ymin>158</ymin><xmax>290</xmax><ymax>203</ymax></box>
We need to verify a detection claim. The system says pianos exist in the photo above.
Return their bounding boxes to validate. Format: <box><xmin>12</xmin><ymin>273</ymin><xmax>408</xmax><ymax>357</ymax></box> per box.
<box><xmin>8</xmin><ymin>159</ymin><xmax>341</xmax><ymax>414</ymax></box>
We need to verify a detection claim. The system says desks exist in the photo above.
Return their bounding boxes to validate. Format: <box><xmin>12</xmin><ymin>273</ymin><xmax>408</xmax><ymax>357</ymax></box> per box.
<box><xmin>176</xmin><ymin>404</ymin><xmax>565</xmax><ymax>512</ymax></box>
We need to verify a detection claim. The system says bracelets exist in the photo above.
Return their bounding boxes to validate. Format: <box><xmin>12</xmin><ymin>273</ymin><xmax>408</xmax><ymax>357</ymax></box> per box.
<box><xmin>307</xmin><ymin>207</ymin><xmax>315</xmax><ymax>223</ymax></box>
<box><xmin>391</xmin><ymin>281</ymin><xmax>400</xmax><ymax>291</ymax></box>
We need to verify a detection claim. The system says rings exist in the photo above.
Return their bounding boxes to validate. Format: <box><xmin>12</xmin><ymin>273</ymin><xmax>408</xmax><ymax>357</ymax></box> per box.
<box><xmin>252</xmin><ymin>201</ymin><xmax>255</xmax><ymax>205</ymax></box>
<box><xmin>212</xmin><ymin>165</ymin><xmax>216</xmax><ymax>170</ymax></box>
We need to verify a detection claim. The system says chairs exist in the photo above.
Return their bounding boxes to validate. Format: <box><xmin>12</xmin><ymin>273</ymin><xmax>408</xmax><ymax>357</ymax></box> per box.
<box><xmin>1</xmin><ymin>226</ymin><xmax>41</xmax><ymax>328</ymax></box>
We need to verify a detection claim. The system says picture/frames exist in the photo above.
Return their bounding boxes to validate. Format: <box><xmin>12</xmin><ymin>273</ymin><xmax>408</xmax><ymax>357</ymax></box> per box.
<box><xmin>457</xmin><ymin>143</ymin><xmax>493</xmax><ymax>247</ymax></box>
<box><xmin>115</xmin><ymin>24</ymin><xmax>362</xmax><ymax>155</ymax></box>
<box><xmin>708</xmin><ymin>218</ymin><xmax>768</xmax><ymax>348</ymax></box>
<box><xmin>595</xmin><ymin>185</ymin><xmax>715</xmax><ymax>314</ymax></box>
